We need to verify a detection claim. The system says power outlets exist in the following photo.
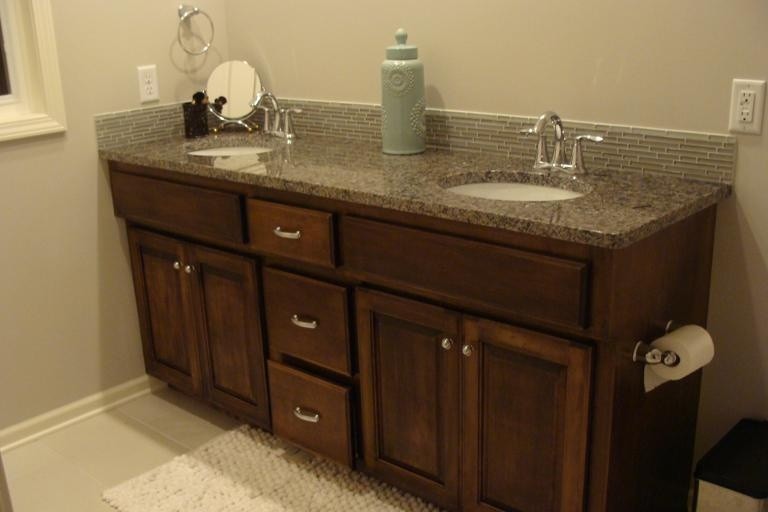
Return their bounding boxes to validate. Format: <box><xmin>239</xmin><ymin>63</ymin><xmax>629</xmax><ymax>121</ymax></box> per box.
<box><xmin>728</xmin><ymin>77</ymin><xmax>766</xmax><ymax>136</ymax></box>
<box><xmin>137</xmin><ymin>65</ymin><xmax>160</xmax><ymax>103</ymax></box>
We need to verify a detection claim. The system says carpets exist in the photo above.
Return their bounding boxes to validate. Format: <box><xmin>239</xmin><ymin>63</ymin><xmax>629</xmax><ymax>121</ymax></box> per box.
<box><xmin>101</xmin><ymin>424</ymin><xmax>440</xmax><ymax>512</ymax></box>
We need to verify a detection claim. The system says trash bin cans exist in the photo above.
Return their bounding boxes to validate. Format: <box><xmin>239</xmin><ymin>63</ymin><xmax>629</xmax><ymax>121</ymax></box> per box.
<box><xmin>694</xmin><ymin>417</ymin><xmax>768</xmax><ymax>512</ymax></box>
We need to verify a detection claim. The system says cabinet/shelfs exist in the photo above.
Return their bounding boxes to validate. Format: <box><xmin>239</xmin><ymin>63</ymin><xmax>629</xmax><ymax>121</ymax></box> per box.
<box><xmin>243</xmin><ymin>189</ymin><xmax>359</xmax><ymax>468</ymax></box>
<box><xmin>340</xmin><ymin>204</ymin><xmax>718</xmax><ymax>512</ymax></box>
<box><xmin>107</xmin><ymin>168</ymin><xmax>271</xmax><ymax>432</ymax></box>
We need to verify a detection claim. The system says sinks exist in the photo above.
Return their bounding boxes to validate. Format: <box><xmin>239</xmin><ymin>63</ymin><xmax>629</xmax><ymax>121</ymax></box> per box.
<box><xmin>438</xmin><ymin>170</ymin><xmax>594</xmax><ymax>202</ymax></box>
<box><xmin>187</xmin><ymin>146</ymin><xmax>273</xmax><ymax>156</ymax></box>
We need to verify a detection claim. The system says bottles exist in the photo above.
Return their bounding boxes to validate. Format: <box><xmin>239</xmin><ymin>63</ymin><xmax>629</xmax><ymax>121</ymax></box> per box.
<box><xmin>380</xmin><ymin>27</ymin><xmax>427</xmax><ymax>155</ymax></box>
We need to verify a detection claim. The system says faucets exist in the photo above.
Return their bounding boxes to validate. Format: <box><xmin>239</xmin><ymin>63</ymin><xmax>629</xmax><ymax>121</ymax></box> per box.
<box><xmin>249</xmin><ymin>90</ymin><xmax>282</xmax><ymax>131</ymax></box>
<box><xmin>545</xmin><ymin>201</ymin><xmax>562</xmax><ymax>237</ymax></box>
<box><xmin>271</xmin><ymin>141</ymin><xmax>288</xmax><ymax>185</ymax></box>
<box><xmin>533</xmin><ymin>110</ymin><xmax>568</xmax><ymax>166</ymax></box>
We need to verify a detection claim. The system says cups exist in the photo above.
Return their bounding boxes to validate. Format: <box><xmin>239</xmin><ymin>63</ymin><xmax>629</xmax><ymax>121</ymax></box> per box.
<box><xmin>182</xmin><ymin>102</ymin><xmax>210</xmax><ymax>139</ymax></box>
